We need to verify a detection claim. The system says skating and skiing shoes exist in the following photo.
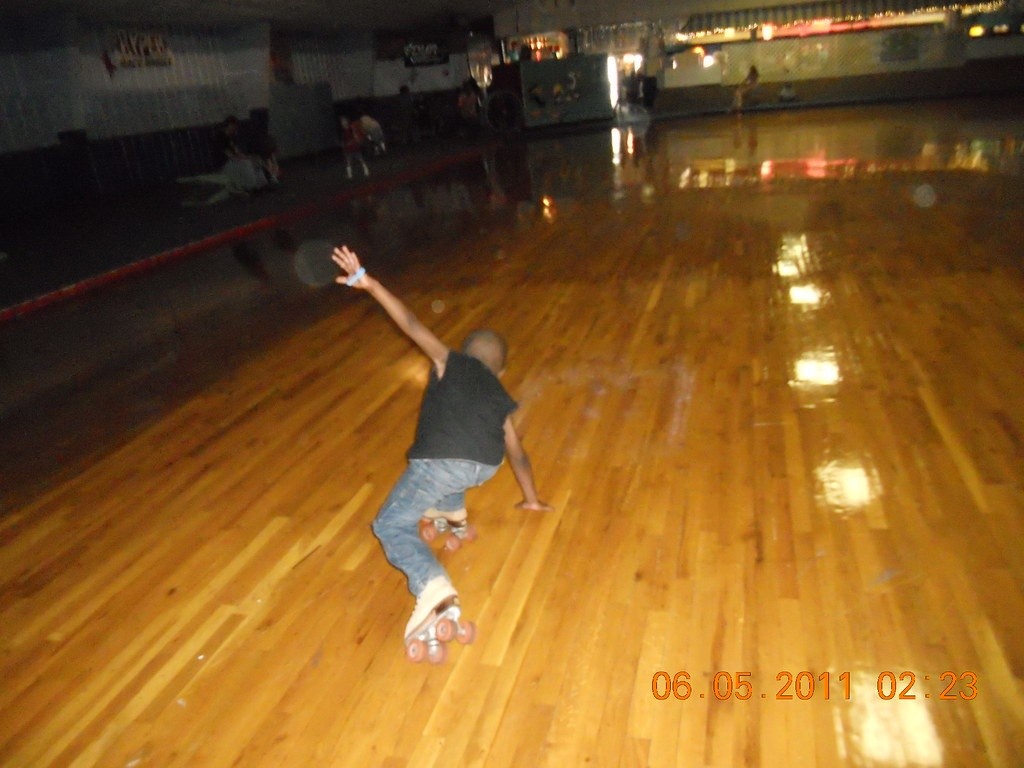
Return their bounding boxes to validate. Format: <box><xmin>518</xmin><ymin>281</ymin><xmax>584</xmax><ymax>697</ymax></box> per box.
<box><xmin>402</xmin><ymin>578</ymin><xmax>475</xmax><ymax>664</ymax></box>
<box><xmin>421</xmin><ymin>508</ymin><xmax>477</xmax><ymax>551</ymax></box>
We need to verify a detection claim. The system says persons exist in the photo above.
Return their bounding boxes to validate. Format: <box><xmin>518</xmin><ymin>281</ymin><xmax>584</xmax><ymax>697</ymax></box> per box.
<box><xmin>457</xmin><ymin>78</ymin><xmax>483</xmax><ymax>145</ymax></box>
<box><xmin>777</xmin><ymin>81</ymin><xmax>801</xmax><ymax>103</ymax></box>
<box><xmin>361</xmin><ymin>114</ymin><xmax>388</xmax><ymax>156</ymax></box>
<box><xmin>616</xmin><ymin>63</ymin><xmax>658</xmax><ymax>124</ymax></box>
<box><xmin>340</xmin><ymin>110</ymin><xmax>370</xmax><ymax>181</ymax></box>
<box><xmin>331</xmin><ymin>245</ymin><xmax>556</xmax><ymax>665</ymax></box>
<box><xmin>212</xmin><ymin>116</ymin><xmax>248</xmax><ymax>172</ymax></box>
<box><xmin>176</xmin><ymin>136</ymin><xmax>281</xmax><ymax>209</ymax></box>
<box><xmin>399</xmin><ymin>86</ymin><xmax>445</xmax><ymax>144</ymax></box>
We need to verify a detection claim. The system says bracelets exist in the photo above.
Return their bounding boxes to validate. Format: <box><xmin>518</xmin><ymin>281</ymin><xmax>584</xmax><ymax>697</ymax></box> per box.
<box><xmin>346</xmin><ymin>267</ymin><xmax>366</xmax><ymax>287</ymax></box>
